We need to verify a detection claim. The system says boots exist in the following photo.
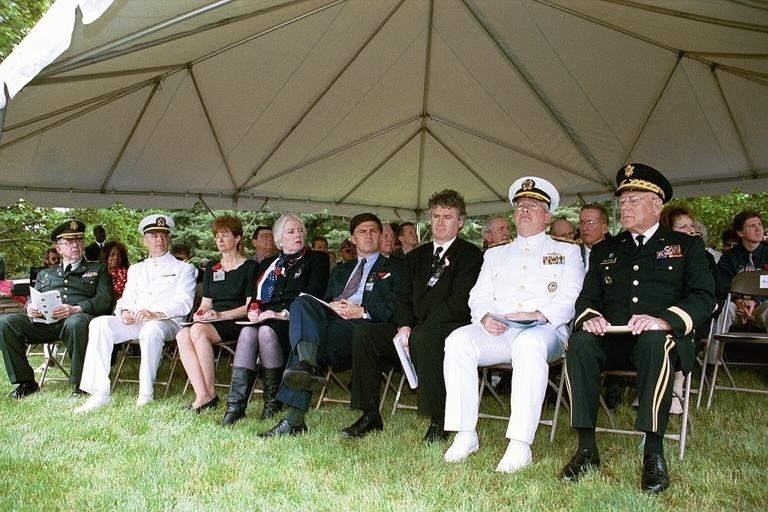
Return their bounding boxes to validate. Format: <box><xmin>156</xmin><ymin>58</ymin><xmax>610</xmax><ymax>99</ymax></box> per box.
<box><xmin>223</xmin><ymin>366</ymin><xmax>257</xmax><ymax>425</ymax></box>
<box><xmin>259</xmin><ymin>366</ymin><xmax>284</xmax><ymax>422</ymax></box>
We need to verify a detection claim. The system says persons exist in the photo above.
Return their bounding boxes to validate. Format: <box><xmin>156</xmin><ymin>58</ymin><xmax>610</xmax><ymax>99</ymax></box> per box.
<box><xmin>1</xmin><ymin>162</ymin><xmax>768</xmax><ymax>492</ymax></box>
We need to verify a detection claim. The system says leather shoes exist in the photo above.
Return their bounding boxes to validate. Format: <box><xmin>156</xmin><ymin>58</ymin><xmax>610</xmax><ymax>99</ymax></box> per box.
<box><xmin>557</xmin><ymin>449</ymin><xmax>600</xmax><ymax>482</ymax></box>
<box><xmin>73</xmin><ymin>392</ymin><xmax>110</xmax><ymax>413</ymax></box>
<box><xmin>445</xmin><ymin>432</ymin><xmax>480</xmax><ymax>462</ymax></box>
<box><xmin>496</xmin><ymin>441</ymin><xmax>533</xmax><ymax>472</ymax></box>
<box><xmin>642</xmin><ymin>455</ymin><xmax>669</xmax><ymax>491</ymax></box>
<box><xmin>137</xmin><ymin>392</ymin><xmax>153</xmax><ymax>406</ymax></box>
<box><xmin>7</xmin><ymin>382</ymin><xmax>38</xmax><ymax>400</ymax></box>
<box><xmin>283</xmin><ymin>360</ymin><xmax>328</xmax><ymax>392</ymax></box>
<box><xmin>341</xmin><ymin>412</ymin><xmax>383</xmax><ymax>436</ymax></box>
<box><xmin>422</xmin><ymin>417</ymin><xmax>449</xmax><ymax>441</ymax></box>
<box><xmin>256</xmin><ymin>419</ymin><xmax>307</xmax><ymax>436</ymax></box>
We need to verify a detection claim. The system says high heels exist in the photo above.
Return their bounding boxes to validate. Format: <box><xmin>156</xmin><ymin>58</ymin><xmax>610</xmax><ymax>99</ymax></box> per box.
<box><xmin>188</xmin><ymin>400</ymin><xmax>215</xmax><ymax>414</ymax></box>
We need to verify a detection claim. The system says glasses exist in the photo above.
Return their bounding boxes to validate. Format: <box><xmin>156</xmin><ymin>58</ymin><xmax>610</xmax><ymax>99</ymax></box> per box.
<box><xmin>58</xmin><ymin>239</ymin><xmax>83</xmax><ymax>247</ymax></box>
<box><xmin>257</xmin><ymin>234</ymin><xmax>272</xmax><ymax>239</ymax></box>
<box><xmin>724</xmin><ymin>243</ymin><xmax>737</xmax><ymax>248</ymax></box>
<box><xmin>513</xmin><ymin>202</ymin><xmax>544</xmax><ymax>210</ymax></box>
<box><xmin>614</xmin><ymin>195</ymin><xmax>645</xmax><ymax>206</ymax></box>
<box><xmin>343</xmin><ymin>249</ymin><xmax>354</xmax><ymax>253</ymax></box>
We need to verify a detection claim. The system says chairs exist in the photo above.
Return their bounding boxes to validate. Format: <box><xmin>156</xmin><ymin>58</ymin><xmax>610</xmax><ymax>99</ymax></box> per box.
<box><xmin>706</xmin><ymin>269</ymin><xmax>768</xmax><ymax>411</ymax></box>
<box><xmin>24</xmin><ymin>330</ymin><xmax>266</xmax><ymax>403</ymax></box>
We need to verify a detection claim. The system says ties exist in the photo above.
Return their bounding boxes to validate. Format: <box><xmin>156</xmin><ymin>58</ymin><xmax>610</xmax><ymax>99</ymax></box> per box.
<box><xmin>636</xmin><ymin>235</ymin><xmax>644</xmax><ymax>247</ymax></box>
<box><xmin>432</xmin><ymin>247</ymin><xmax>442</xmax><ymax>275</ymax></box>
<box><xmin>337</xmin><ymin>258</ymin><xmax>367</xmax><ymax>301</ymax></box>
<box><xmin>100</xmin><ymin>243</ymin><xmax>103</xmax><ymax>248</ymax></box>
<box><xmin>746</xmin><ymin>253</ymin><xmax>755</xmax><ymax>272</ymax></box>
<box><xmin>64</xmin><ymin>264</ymin><xmax>72</xmax><ymax>275</ymax></box>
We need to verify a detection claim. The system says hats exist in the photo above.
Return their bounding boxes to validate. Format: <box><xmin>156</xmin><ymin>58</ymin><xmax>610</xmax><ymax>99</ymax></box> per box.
<box><xmin>508</xmin><ymin>176</ymin><xmax>559</xmax><ymax>213</ymax></box>
<box><xmin>138</xmin><ymin>214</ymin><xmax>176</xmax><ymax>235</ymax></box>
<box><xmin>50</xmin><ymin>221</ymin><xmax>86</xmax><ymax>241</ymax></box>
<box><xmin>613</xmin><ymin>164</ymin><xmax>673</xmax><ymax>204</ymax></box>
<box><xmin>340</xmin><ymin>239</ymin><xmax>355</xmax><ymax>248</ymax></box>
